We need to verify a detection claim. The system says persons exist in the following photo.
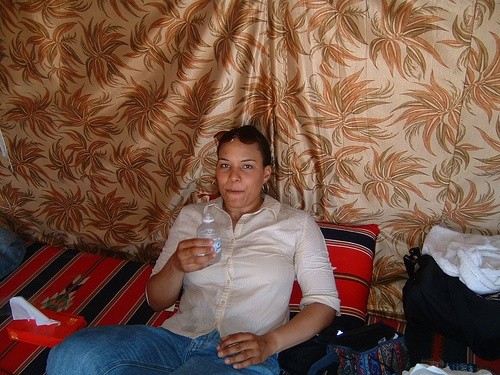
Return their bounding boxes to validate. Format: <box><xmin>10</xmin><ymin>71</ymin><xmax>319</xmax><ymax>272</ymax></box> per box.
<box><xmin>46</xmin><ymin>125</ymin><xmax>340</xmax><ymax>375</ymax></box>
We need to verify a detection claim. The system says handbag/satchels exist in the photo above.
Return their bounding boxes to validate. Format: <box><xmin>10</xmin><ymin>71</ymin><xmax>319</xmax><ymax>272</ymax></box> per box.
<box><xmin>308</xmin><ymin>322</ymin><xmax>409</xmax><ymax>374</ymax></box>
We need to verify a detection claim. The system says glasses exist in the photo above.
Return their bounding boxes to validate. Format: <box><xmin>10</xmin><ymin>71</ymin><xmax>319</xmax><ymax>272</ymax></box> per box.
<box><xmin>213</xmin><ymin>126</ymin><xmax>266</xmax><ymax>151</ymax></box>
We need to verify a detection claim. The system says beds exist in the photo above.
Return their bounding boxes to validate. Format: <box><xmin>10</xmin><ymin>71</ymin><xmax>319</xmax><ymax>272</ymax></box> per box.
<box><xmin>0</xmin><ymin>236</ymin><xmax>500</xmax><ymax>375</ymax></box>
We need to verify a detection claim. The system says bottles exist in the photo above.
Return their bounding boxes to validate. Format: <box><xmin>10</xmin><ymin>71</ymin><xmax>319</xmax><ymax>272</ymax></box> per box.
<box><xmin>197</xmin><ymin>211</ymin><xmax>222</xmax><ymax>263</ymax></box>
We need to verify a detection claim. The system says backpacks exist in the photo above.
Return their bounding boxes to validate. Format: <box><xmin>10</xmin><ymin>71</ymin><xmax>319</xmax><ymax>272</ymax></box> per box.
<box><xmin>400</xmin><ymin>247</ymin><xmax>500</xmax><ymax>363</ymax></box>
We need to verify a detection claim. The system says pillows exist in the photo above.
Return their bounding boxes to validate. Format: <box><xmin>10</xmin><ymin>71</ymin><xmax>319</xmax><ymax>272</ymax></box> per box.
<box><xmin>289</xmin><ymin>220</ymin><xmax>383</xmax><ymax>350</ymax></box>
